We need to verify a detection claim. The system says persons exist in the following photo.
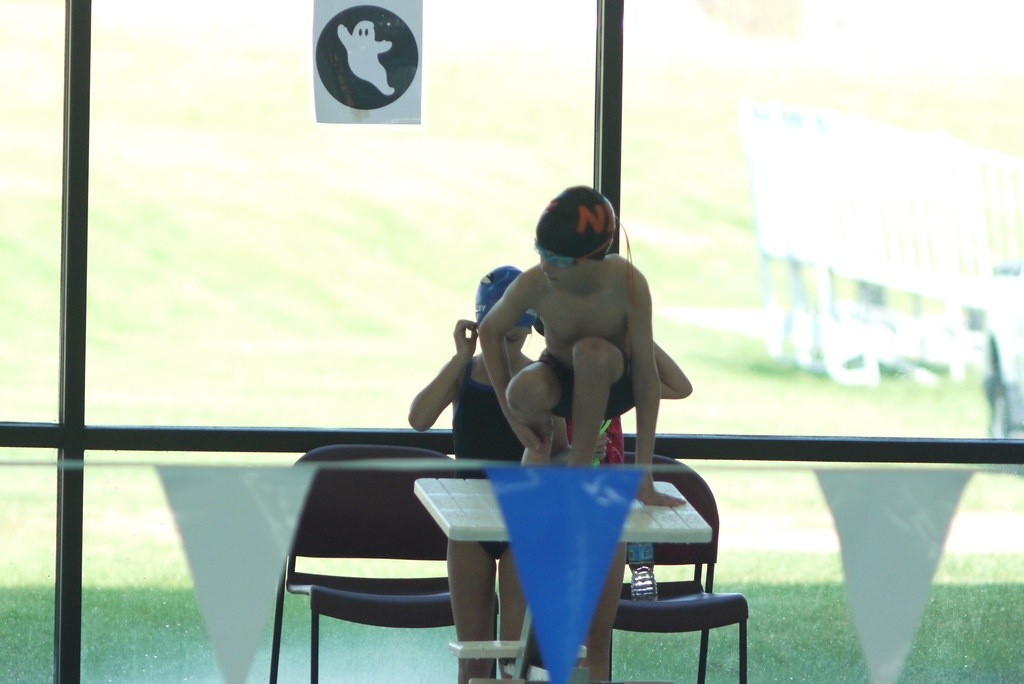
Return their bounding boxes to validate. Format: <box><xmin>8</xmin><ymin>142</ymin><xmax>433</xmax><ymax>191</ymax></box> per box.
<box><xmin>478</xmin><ymin>186</ymin><xmax>688</xmax><ymax>509</ymax></box>
<box><xmin>408</xmin><ymin>263</ymin><xmax>694</xmax><ymax>684</ymax></box>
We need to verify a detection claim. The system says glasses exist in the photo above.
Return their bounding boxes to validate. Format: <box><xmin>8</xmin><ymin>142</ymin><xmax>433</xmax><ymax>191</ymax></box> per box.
<box><xmin>535</xmin><ymin>235</ymin><xmax>613</xmax><ymax>270</ymax></box>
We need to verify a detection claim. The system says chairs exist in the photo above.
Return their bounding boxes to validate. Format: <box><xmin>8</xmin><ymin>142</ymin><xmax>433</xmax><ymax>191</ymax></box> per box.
<box><xmin>610</xmin><ymin>451</ymin><xmax>748</xmax><ymax>684</ymax></box>
<box><xmin>269</xmin><ymin>444</ymin><xmax>499</xmax><ymax>684</ymax></box>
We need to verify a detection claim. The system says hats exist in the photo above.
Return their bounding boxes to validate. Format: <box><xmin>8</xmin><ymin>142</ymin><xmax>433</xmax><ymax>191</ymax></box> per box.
<box><xmin>537</xmin><ymin>185</ymin><xmax>615</xmax><ymax>261</ymax></box>
<box><xmin>475</xmin><ymin>266</ymin><xmax>537</xmax><ymax>329</ymax></box>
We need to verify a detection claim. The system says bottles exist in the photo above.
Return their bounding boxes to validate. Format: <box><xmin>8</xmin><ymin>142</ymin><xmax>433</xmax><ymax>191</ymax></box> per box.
<box><xmin>626</xmin><ymin>542</ymin><xmax>658</xmax><ymax>602</ymax></box>
